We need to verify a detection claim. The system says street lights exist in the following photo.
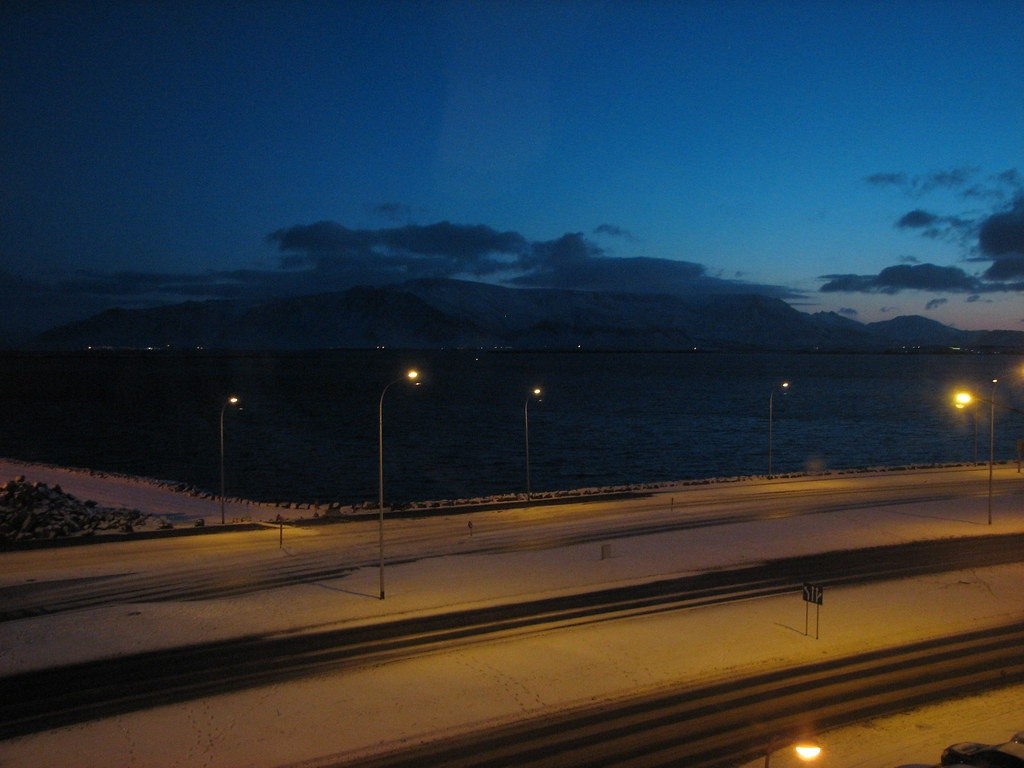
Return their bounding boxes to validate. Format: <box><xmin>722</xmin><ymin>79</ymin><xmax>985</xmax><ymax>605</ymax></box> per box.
<box><xmin>525</xmin><ymin>389</ymin><xmax>541</xmax><ymax>507</ymax></box>
<box><xmin>220</xmin><ymin>397</ymin><xmax>239</xmax><ymax>524</ymax></box>
<box><xmin>380</xmin><ymin>372</ymin><xmax>419</xmax><ymax>600</ymax></box>
<box><xmin>954</xmin><ymin>392</ymin><xmax>993</xmax><ymax>527</ymax></box>
<box><xmin>768</xmin><ymin>383</ymin><xmax>789</xmax><ymax>476</ymax></box>
